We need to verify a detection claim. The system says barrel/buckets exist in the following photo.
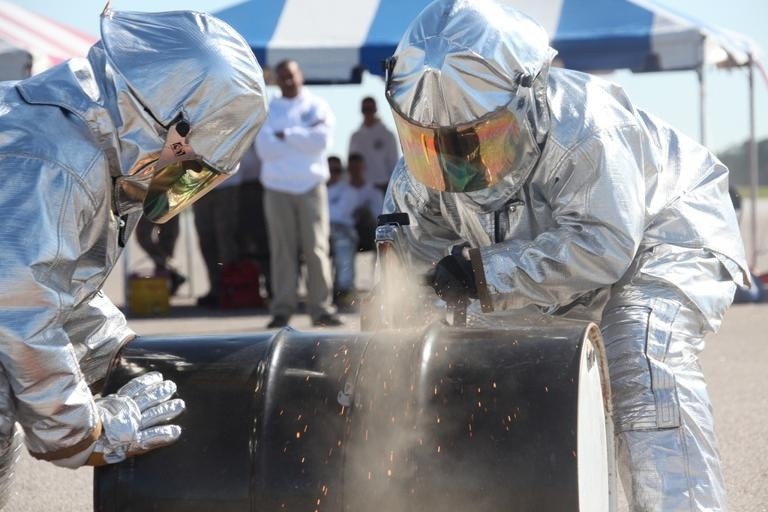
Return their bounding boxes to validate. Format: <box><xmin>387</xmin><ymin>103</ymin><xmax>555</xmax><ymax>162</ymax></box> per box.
<box><xmin>93</xmin><ymin>314</ymin><xmax>618</xmax><ymax>512</ymax></box>
<box><xmin>128</xmin><ymin>270</ymin><xmax>171</xmax><ymax>315</ymax></box>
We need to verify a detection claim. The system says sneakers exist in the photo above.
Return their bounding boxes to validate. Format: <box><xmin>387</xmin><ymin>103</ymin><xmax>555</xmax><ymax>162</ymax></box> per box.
<box><xmin>267</xmin><ymin>313</ymin><xmax>342</xmax><ymax>328</ymax></box>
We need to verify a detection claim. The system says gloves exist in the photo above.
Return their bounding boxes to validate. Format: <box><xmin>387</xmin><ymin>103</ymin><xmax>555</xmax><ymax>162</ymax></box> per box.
<box><xmin>431</xmin><ymin>241</ymin><xmax>481</xmax><ymax>311</ymax></box>
<box><xmin>90</xmin><ymin>371</ymin><xmax>184</xmax><ymax>466</ymax></box>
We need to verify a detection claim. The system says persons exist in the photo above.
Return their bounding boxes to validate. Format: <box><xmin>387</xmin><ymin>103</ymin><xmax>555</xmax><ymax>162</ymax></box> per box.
<box><xmin>0</xmin><ymin>2</ymin><xmax>269</xmax><ymax>510</ymax></box>
<box><xmin>373</xmin><ymin>0</ymin><xmax>750</xmax><ymax>512</ymax></box>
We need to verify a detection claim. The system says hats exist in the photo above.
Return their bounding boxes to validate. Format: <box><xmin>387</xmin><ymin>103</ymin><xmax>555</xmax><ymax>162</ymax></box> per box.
<box><xmin>381</xmin><ymin>0</ymin><xmax>558</xmax><ymax>216</ymax></box>
<box><xmin>80</xmin><ymin>8</ymin><xmax>269</xmax><ymax>227</ymax></box>
